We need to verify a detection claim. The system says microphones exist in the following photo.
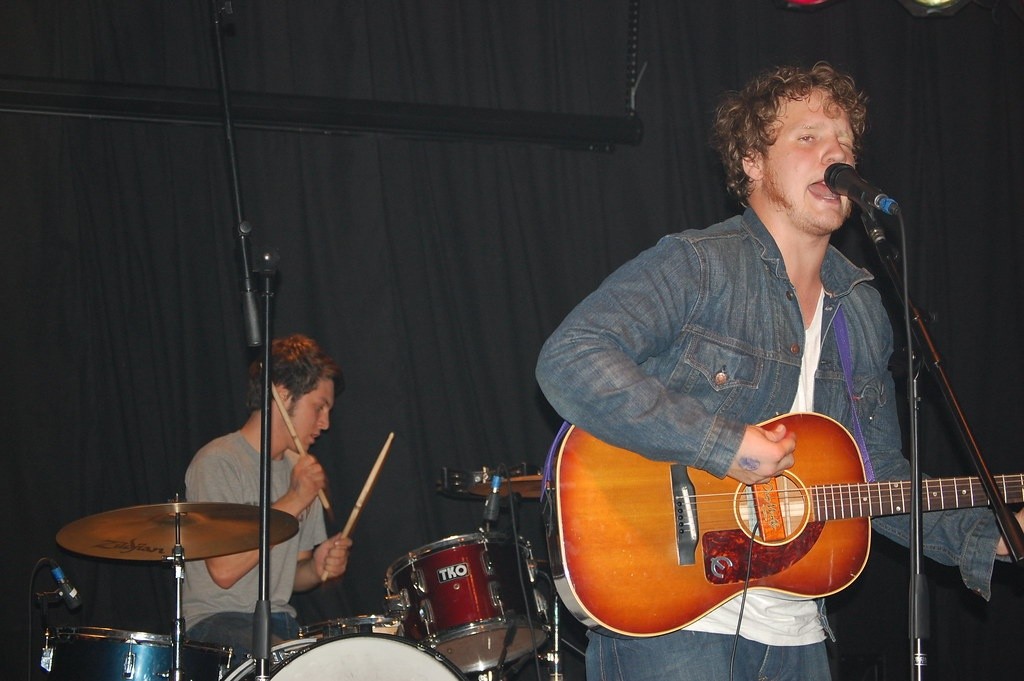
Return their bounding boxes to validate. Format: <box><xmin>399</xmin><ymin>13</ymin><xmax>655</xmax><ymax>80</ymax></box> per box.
<box><xmin>824</xmin><ymin>163</ymin><xmax>900</xmax><ymax>215</ymax></box>
<box><xmin>50</xmin><ymin>559</ymin><xmax>83</xmax><ymax>610</ymax></box>
<box><xmin>484</xmin><ymin>464</ymin><xmax>504</xmax><ymax>520</ymax></box>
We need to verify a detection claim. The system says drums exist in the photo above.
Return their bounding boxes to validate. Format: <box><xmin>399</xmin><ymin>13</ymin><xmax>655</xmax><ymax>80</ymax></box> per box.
<box><xmin>39</xmin><ymin>625</ymin><xmax>236</xmax><ymax>681</ymax></box>
<box><xmin>218</xmin><ymin>614</ymin><xmax>470</xmax><ymax>681</ymax></box>
<box><xmin>384</xmin><ymin>529</ymin><xmax>551</xmax><ymax>674</ymax></box>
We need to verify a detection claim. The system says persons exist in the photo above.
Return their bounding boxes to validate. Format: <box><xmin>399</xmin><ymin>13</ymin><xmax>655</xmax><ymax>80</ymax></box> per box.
<box><xmin>534</xmin><ymin>61</ymin><xmax>1024</xmax><ymax>681</ymax></box>
<box><xmin>183</xmin><ymin>334</ymin><xmax>353</xmax><ymax>665</ymax></box>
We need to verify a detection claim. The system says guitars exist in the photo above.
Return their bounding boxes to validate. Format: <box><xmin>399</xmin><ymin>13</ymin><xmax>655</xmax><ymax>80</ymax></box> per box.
<box><xmin>539</xmin><ymin>409</ymin><xmax>1024</xmax><ymax>640</ymax></box>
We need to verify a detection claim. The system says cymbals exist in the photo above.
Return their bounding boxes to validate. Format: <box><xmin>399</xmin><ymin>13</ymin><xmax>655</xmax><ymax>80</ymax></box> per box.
<box><xmin>467</xmin><ymin>473</ymin><xmax>553</xmax><ymax>499</ymax></box>
<box><xmin>54</xmin><ymin>501</ymin><xmax>301</xmax><ymax>561</ymax></box>
